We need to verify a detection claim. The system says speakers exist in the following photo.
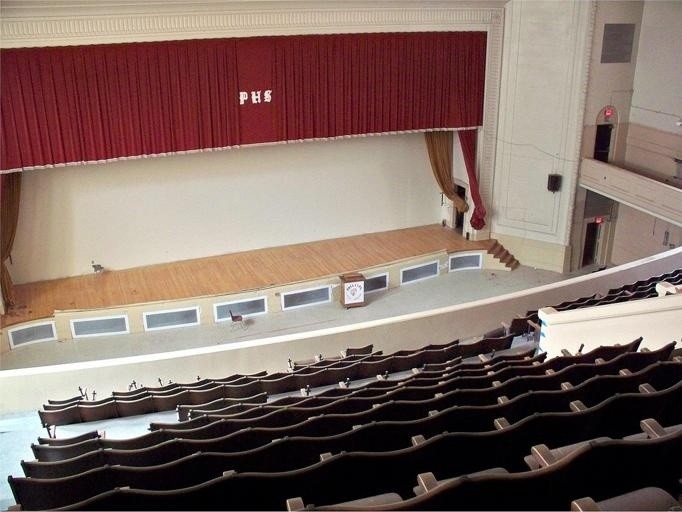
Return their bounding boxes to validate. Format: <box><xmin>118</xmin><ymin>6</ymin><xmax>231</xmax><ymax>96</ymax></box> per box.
<box><xmin>548</xmin><ymin>174</ymin><xmax>561</xmax><ymax>191</ymax></box>
